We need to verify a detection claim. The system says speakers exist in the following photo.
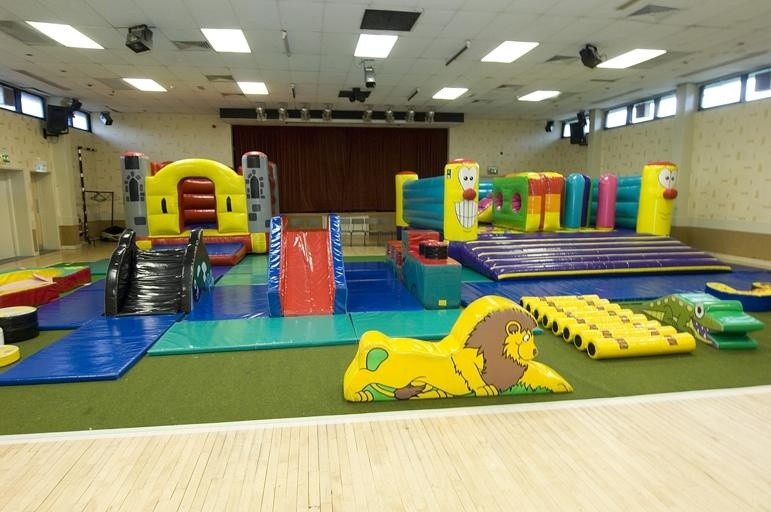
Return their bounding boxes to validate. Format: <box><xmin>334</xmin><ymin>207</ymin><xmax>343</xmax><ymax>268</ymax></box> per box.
<box><xmin>570</xmin><ymin>123</ymin><xmax>583</xmax><ymax>144</ymax></box>
<box><xmin>47</xmin><ymin>104</ymin><xmax>67</xmax><ymax>131</ymax></box>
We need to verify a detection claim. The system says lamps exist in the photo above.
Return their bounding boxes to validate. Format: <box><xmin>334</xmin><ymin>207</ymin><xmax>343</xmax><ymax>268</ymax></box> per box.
<box><xmin>364</xmin><ymin>66</ymin><xmax>377</xmax><ymax>88</ymax></box>
<box><xmin>255</xmin><ymin>102</ymin><xmax>436</xmax><ymax>126</ymax></box>
<box><xmin>578</xmin><ymin>43</ymin><xmax>602</xmax><ymax>69</ymax></box>
<box><xmin>125</xmin><ymin>24</ymin><xmax>153</xmax><ymax>54</ymax></box>
<box><xmin>67</xmin><ymin>98</ymin><xmax>82</xmax><ymax>111</ymax></box>
<box><xmin>99</xmin><ymin>111</ymin><xmax>113</xmax><ymax>125</ymax></box>
<box><xmin>577</xmin><ymin>111</ymin><xmax>586</xmax><ymax>126</ymax></box>
<box><xmin>544</xmin><ymin>120</ymin><xmax>554</xmax><ymax>132</ymax></box>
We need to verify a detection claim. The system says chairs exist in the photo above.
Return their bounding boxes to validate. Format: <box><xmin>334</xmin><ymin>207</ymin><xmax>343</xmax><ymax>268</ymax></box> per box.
<box><xmin>340</xmin><ymin>217</ymin><xmax>397</xmax><ymax>248</ymax></box>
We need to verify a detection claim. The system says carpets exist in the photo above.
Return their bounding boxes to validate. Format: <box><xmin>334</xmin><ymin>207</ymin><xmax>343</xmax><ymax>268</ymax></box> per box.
<box><xmin>0</xmin><ymin>254</ymin><xmax>769</xmax><ymax>445</ymax></box>
